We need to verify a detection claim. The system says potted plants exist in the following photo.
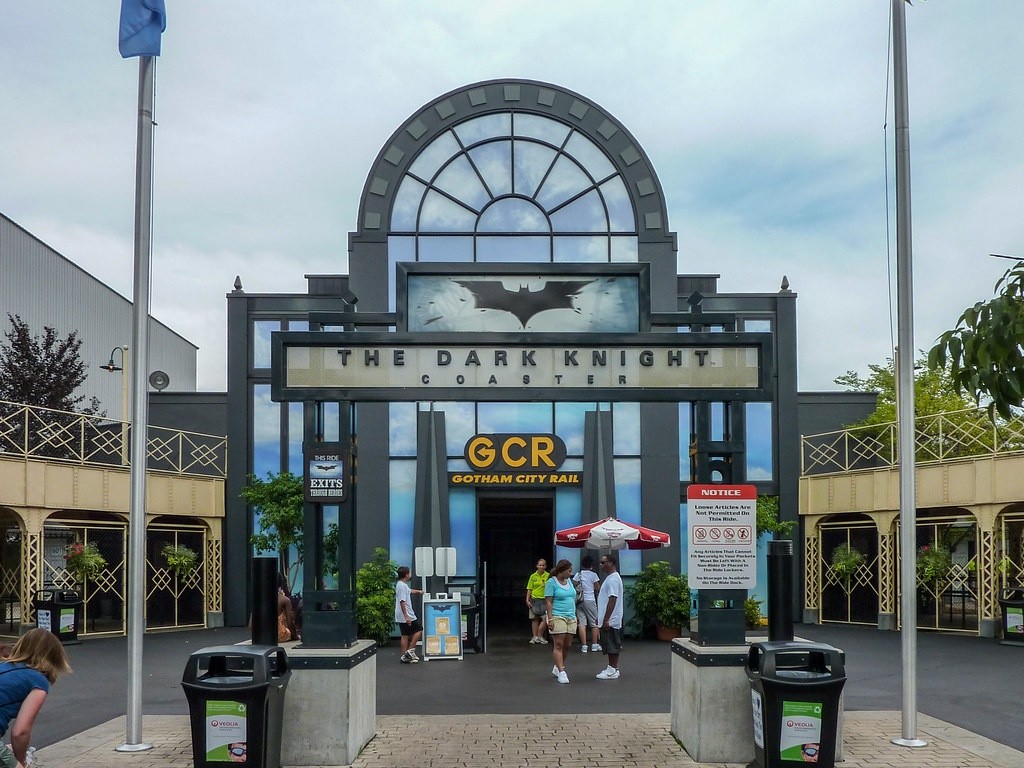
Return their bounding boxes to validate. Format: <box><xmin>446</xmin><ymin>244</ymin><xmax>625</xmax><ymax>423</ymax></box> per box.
<box><xmin>626</xmin><ymin>560</ymin><xmax>690</xmax><ymax>640</ymax></box>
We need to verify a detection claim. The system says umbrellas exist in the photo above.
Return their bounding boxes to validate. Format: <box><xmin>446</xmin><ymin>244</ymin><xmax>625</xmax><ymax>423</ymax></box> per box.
<box><xmin>554</xmin><ymin>516</ymin><xmax>670</xmax><ymax>555</ymax></box>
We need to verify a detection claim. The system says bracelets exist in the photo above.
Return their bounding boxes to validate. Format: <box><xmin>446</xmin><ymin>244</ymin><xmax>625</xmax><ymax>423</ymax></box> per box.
<box><xmin>548</xmin><ymin>619</ymin><xmax>553</xmax><ymax>622</ymax></box>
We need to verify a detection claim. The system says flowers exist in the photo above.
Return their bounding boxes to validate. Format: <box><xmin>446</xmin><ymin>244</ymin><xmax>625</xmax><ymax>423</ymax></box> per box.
<box><xmin>60</xmin><ymin>541</ymin><xmax>109</xmax><ymax>583</ymax></box>
<box><xmin>916</xmin><ymin>545</ymin><xmax>949</xmax><ymax>589</ymax></box>
<box><xmin>163</xmin><ymin>544</ymin><xmax>202</xmax><ymax>575</ymax></box>
<box><xmin>834</xmin><ymin>546</ymin><xmax>869</xmax><ymax>573</ymax></box>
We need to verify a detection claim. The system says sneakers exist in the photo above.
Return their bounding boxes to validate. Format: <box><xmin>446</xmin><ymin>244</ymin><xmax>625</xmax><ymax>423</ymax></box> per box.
<box><xmin>591</xmin><ymin>644</ymin><xmax>602</xmax><ymax>651</ymax></box>
<box><xmin>401</xmin><ymin>653</ymin><xmax>418</xmax><ymax>663</ymax></box>
<box><xmin>558</xmin><ymin>671</ymin><xmax>569</xmax><ymax>684</ymax></box>
<box><xmin>581</xmin><ymin>644</ymin><xmax>587</xmax><ymax>653</ymax></box>
<box><xmin>407</xmin><ymin>648</ymin><xmax>420</xmax><ymax>661</ymax></box>
<box><xmin>595</xmin><ymin>665</ymin><xmax>620</xmax><ymax>678</ymax></box>
<box><xmin>534</xmin><ymin>636</ymin><xmax>549</xmax><ymax>645</ymax></box>
<box><xmin>552</xmin><ymin>664</ymin><xmax>559</xmax><ymax>676</ymax></box>
<box><xmin>529</xmin><ymin>638</ymin><xmax>535</xmax><ymax>644</ymax></box>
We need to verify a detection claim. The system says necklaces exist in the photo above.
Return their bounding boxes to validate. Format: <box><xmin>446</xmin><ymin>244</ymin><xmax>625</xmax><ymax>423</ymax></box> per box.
<box><xmin>278</xmin><ymin>587</ymin><xmax>285</xmax><ymax>595</ymax></box>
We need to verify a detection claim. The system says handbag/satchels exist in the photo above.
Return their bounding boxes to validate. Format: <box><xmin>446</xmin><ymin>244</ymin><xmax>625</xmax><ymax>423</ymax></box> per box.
<box><xmin>530</xmin><ymin>598</ymin><xmax>548</xmax><ymax>615</ymax></box>
<box><xmin>575</xmin><ymin>572</ymin><xmax>584</xmax><ymax>603</ymax></box>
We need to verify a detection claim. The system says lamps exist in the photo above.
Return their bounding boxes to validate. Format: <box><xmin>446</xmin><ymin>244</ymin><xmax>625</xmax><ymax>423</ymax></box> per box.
<box><xmin>686</xmin><ymin>290</ymin><xmax>705</xmax><ymax>311</ymax></box>
<box><xmin>341</xmin><ymin>289</ymin><xmax>359</xmax><ymax>314</ymax></box>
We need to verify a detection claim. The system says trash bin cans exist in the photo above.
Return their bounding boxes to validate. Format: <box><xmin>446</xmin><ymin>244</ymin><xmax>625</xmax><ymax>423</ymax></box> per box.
<box><xmin>179</xmin><ymin>645</ymin><xmax>291</xmax><ymax>768</ymax></box>
<box><xmin>450</xmin><ymin>592</ymin><xmax>481</xmax><ymax>649</ymax></box>
<box><xmin>31</xmin><ymin>587</ymin><xmax>80</xmax><ymax>646</ymax></box>
<box><xmin>745</xmin><ymin>641</ymin><xmax>847</xmax><ymax>768</ymax></box>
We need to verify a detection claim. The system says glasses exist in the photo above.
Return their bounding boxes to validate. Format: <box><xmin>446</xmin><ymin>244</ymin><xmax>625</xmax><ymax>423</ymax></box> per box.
<box><xmin>600</xmin><ymin>560</ymin><xmax>610</xmax><ymax>564</ymax></box>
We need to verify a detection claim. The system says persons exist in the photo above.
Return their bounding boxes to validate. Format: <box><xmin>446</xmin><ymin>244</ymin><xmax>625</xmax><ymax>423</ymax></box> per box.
<box><xmin>572</xmin><ymin>556</ymin><xmax>602</xmax><ymax>652</ymax></box>
<box><xmin>597</xmin><ymin>554</ymin><xmax>623</xmax><ymax>679</ymax></box>
<box><xmin>277</xmin><ymin>578</ymin><xmax>300</xmax><ymax>642</ymax></box>
<box><xmin>0</xmin><ymin>628</ymin><xmax>73</xmax><ymax>768</ymax></box>
<box><xmin>395</xmin><ymin>567</ymin><xmax>424</xmax><ymax>663</ymax></box>
<box><xmin>526</xmin><ymin>559</ymin><xmax>550</xmax><ymax>645</ymax></box>
<box><xmin>544</xmin><ymin>560</ymin><xmax>578</xmax><ymax>684</ymax></box>
